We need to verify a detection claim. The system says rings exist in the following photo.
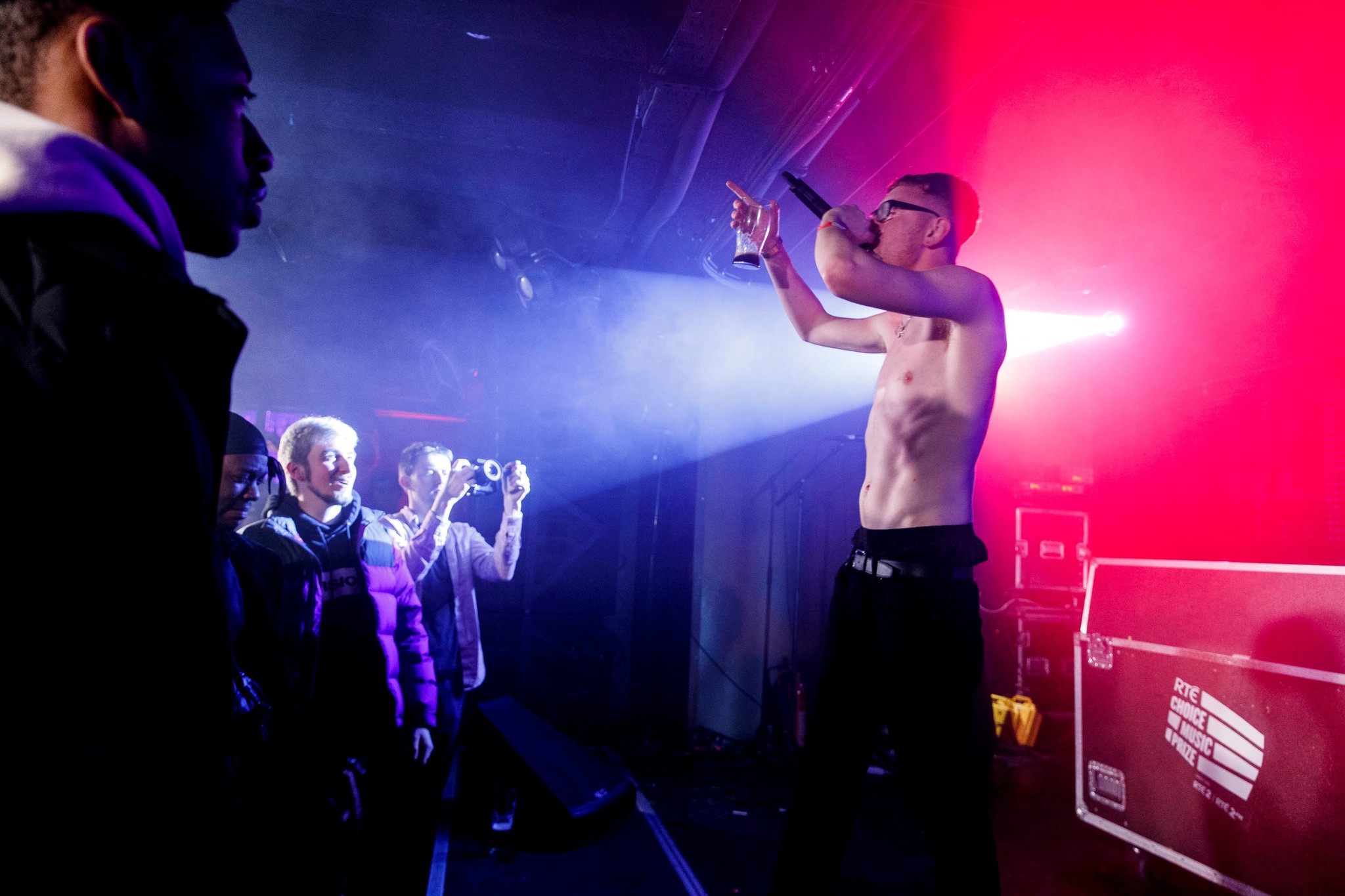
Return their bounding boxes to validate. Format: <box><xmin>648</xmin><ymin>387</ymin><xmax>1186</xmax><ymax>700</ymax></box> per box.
<box><xmin>520</xmin><ymin>470</ymin><xmax>523</xmax><ymax>475</ymax></box>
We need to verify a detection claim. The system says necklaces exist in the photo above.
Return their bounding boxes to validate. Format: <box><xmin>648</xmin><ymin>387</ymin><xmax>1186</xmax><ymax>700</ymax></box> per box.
<box><xmin>893</xmin><ymin>316</ymin><xmax>912</xmax><ymax>338</ymax></box>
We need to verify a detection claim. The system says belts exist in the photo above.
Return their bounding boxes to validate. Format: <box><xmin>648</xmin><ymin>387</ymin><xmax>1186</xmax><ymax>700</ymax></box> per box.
<box><xmin>846</xmin><ymin>548</ymin><xmax>973</xmax><ymax>579</ymax></box>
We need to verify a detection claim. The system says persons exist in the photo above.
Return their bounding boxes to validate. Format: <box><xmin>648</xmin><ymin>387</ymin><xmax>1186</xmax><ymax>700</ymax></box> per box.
<box><xmin>0</xmin><ymin>0</ymin><xmax>276</xmax><ymax>896</ymax></box>
<box><xmin>218</xmin><ymin>412</ymin><xmax>530</xmax><ymax>896</ymax></box>
<box><xmin>726</xmin><ymin>173</ymin><xmax>1007</xmax><ymax>896</ymax></box>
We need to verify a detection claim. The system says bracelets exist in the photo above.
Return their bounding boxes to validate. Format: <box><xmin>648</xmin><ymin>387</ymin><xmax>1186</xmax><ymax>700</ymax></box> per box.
<box><xmin>761</xmin><ymin>235</ymin><xmax>783</xmax><ymax>260</ymax></box>
<box><xmin>816</xmin><ymin>220</ymin><xmax>846</xmax><ymax>231</ymax></box>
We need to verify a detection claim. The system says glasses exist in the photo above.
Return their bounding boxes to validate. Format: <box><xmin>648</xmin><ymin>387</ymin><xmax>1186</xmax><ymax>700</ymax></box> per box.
<box><xmin>868</xmin><ymin>199</ymin><xmax>939</xmax><ymax>222</ymax></box>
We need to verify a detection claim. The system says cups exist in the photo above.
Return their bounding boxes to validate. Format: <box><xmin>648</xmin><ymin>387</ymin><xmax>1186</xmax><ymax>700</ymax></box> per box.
<box><xmin>732</xmin><ymin>196</ymin><xmax>772</xmax><ymax>269</ymax></box>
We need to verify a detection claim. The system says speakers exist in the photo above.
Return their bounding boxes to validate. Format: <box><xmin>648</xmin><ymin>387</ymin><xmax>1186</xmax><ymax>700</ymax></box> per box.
<box><xmin>451</xmin><ymin>695</ymin><xmax>636</xmax><ymax>854</ymax></box>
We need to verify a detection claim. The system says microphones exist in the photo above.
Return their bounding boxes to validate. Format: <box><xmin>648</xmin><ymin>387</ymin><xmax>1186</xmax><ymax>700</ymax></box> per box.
<box><xmin>779</xmin><ymin>168</ymin><xmax>873</xmax><ymax>255</ymax></box>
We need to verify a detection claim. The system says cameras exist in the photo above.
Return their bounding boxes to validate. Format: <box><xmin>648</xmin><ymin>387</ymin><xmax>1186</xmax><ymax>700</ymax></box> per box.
<box><xmin>462</xmin><ymin>456</ymin><xmax>505</xmax><ymax>498</ymax></box>
